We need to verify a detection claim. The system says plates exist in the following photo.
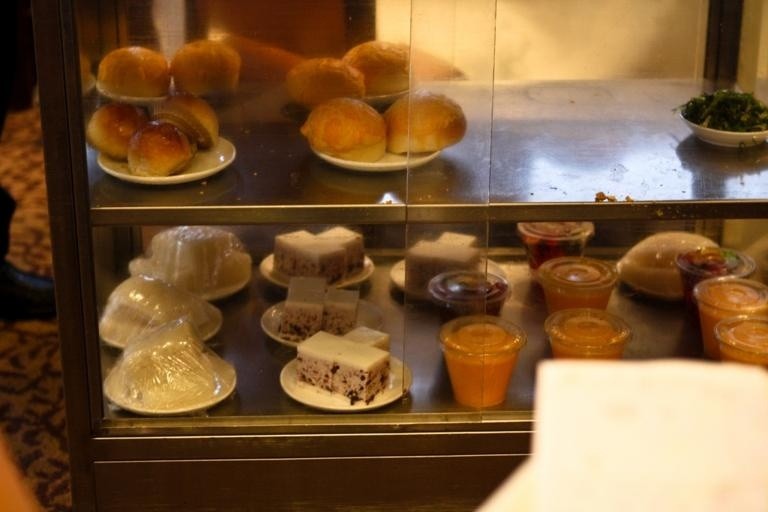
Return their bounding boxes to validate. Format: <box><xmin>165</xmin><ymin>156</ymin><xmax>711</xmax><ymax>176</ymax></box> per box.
<box><xmin>306</xmin><ymin>166</ymin><xmax>439</xmax><ymax>197</ymax></box>
<box><xmin>90</xmin><ymin>76</ymin><xmax>173</xmax><ymax>110</ymax></box>
<box><xmin>336</xmin><ymin>78</ymin><xmax>415</xmax><ymax>106</ymax></box>
<box><xmin>257</xmin><ymin>249</ymin><xmax>377</xmax><ymax>289</ymax></box>
<box><xmin>101</xmin><ymin>366</ymin><xmax>239</xmax><ymax>414</ymax></box>
<box><xmin>615</xmin><ymin>231</ymin><xmax>719</xmax><ymax>299</ymax></box>
<box><xmin>96</xmin><ymin>133</ymin><xmax>238</xmax><ymax>187</ymax></box>
<box><xmin>677</xmin><ymin>109</ymin><xmax>768</xmax><ymax>152</ymax></box>
<box><xmin>99</xmin><ymin>302</ymin><xmax>232</xmax><ymax>356</ymax></box>
<box><xmin>670</xmin><ymin>132</ymin><xmax>768</xmax><ymax>175</ymax></box>
<box><xmin>388</xmin><ymin>255</ymin><xmax>509</xmax><ymax>298</ymax></box>
<box><xmin>94</xmin><ymin>173</ymin><xmax>239</xmax><ymax>206</ymax></box>
<box><xmin>276</xmin><ymin>355</ymin><xmax>412</xmax><ymax>413</ymax></box>
<box><xmin>259</xmin><ymin>296</ymin><xmax>388</xmax><ymax>349</ymax></box>
<box><xmin>300</xmin><ymin>135</ymin><xmax>445</xmax><ymax>178</ymax></box>
<box><xmin>196</xmin><ymin>264</ymin><xmax>254</xmax><ymax>301</ymax></box>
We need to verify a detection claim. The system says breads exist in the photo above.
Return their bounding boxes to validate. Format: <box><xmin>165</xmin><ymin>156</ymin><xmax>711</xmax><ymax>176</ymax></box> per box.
<box><xmin>83</xmin><ymin>41</ymin><xmax>242</xmax><ymax>176</ymax></box>
<box><xmin>283</xmin><ymin>39</ymin><xmax>466</xmax><ymax>158</ymax></box>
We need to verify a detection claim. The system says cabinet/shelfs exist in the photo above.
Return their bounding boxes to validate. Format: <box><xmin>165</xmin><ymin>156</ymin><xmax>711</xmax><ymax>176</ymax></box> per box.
<box><xmin>84</xmin><ymin>72</ymin><xmax>768</xmax><ymax>462</ymax></box>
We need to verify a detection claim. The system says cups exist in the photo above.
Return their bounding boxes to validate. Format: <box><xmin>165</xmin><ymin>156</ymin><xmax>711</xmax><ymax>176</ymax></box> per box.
<box><xmin>425</xmin><ymin>268</ymin><xmax>510</xmax><ymax>320</ymax></box>
<box><xmin>538</xmin><ymin>307</ymin><xmax>635</xmax><ymax>358</ymax></box>
<box><xmin>515</xmin><ymin>221</ymin><xmax>598</xmax><ymax>283</ymax></box>
<box><xmin>675</xmin><ymin>245</ymin><xmax>768</xmax><ymax>369</ymax></box>
<box><xmin>437</xmin><ymin>312</ymin><xmax>526</xmax><ymax>408</ymax></box>
<box><xmin>534</xmin><ymin>257</ymin><xmax>620</xmax><ymax>311</ymax></box>
